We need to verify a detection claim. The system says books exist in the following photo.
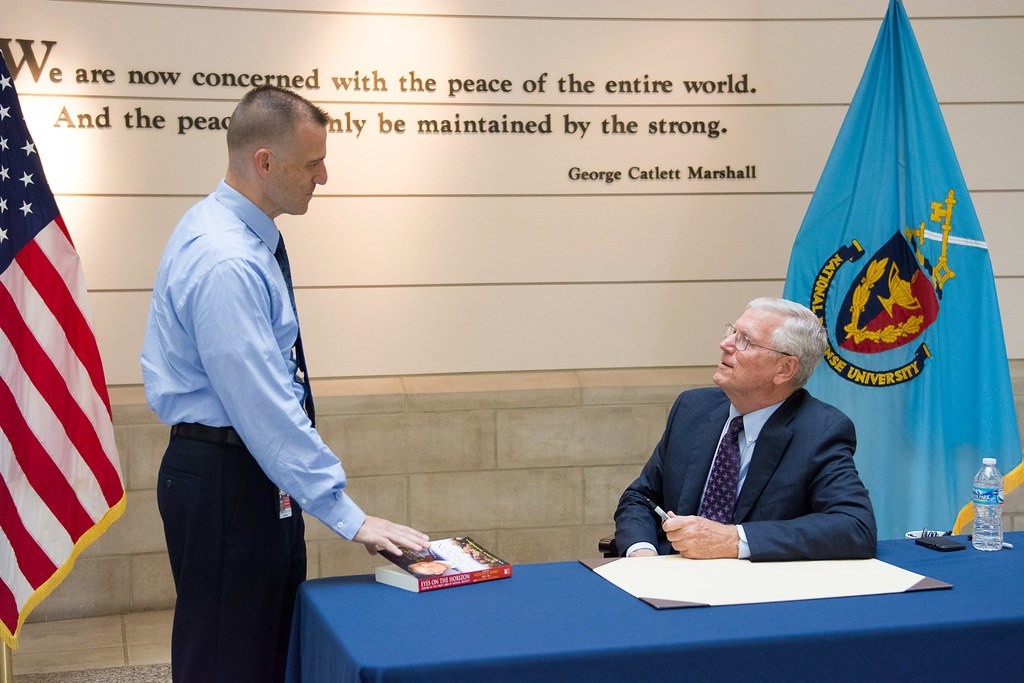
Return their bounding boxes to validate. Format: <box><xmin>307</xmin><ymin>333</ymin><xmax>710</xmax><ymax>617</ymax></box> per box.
<box><xmin>375</xmin><ymin>536</ymin><xmax>512</xmax><ymax>593</ymax></box>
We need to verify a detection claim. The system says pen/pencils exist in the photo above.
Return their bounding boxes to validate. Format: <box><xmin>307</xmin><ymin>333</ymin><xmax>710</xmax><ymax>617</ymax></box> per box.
<box><xmin>642</xmin><ymin>495</ymin><xmax>671</xmax><ymax>521</ymax></box>
<box><xmin>968</xmin><ymin>534</ymin><xmax>1014</xmax><ymax>549</ymax></box>
<box><xmin>921</xmin><ymin>528</ymin><xmax>952</xmax><ymax>538</ymax></box>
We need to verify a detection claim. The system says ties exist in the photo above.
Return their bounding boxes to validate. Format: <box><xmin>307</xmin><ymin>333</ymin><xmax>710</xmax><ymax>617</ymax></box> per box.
<box><xmin>278</xmin><ymin>231</ymin><xmax>316</xmax><ymax>429</ymax></box>
<box><xmin>699</xmin><ymin>416</ymin><xmax>744</xmax><ymax>523</ymax></box>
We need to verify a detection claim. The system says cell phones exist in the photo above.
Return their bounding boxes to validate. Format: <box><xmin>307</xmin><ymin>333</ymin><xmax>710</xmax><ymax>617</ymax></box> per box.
<box><xmin>915</xmin><ymin>536</ymin><xmax>965</xmax><ymax>552</ymax></box>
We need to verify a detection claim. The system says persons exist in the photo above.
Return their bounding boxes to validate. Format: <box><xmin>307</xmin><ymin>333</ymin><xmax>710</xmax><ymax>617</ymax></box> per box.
<box><xmin>613</xmin><ymin>297</ymin><xmax>878</xmax><ymax>563</ymax></box>
<box><xmin>141</xmin><ymin>86</ymin><xmax>432</xmax><ymax>683</ymax></box>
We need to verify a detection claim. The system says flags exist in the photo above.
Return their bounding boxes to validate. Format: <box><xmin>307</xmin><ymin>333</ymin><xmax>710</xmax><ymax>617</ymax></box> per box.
<box><xmin>0</xmin><ymin>49</ymin><xmax>127</xmax><ymax>653</ymax></box>
<box><xmin>782</xmin><ymin>0</ymin><xmax>1023</xmax><ymax>541</ymax></box>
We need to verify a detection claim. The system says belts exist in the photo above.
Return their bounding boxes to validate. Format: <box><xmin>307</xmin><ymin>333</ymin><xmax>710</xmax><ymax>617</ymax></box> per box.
<box><xmin>175</xmin><ymin>423</ymin><xmax>242</xmax><ymax>447</ymax></box>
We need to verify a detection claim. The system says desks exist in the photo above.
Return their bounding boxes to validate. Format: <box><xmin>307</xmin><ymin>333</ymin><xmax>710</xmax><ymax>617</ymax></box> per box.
<box><xmin>284</xmin><ymin>531</ymin><xmax>1024</xmax><ymax>683</ymax></box>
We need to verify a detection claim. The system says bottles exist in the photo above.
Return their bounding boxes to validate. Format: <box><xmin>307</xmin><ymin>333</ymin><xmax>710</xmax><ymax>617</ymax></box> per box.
<box><xmin>972</xmin><ymin>458</ymin><xmax>1004</xmax><ymax>551</ymax></box>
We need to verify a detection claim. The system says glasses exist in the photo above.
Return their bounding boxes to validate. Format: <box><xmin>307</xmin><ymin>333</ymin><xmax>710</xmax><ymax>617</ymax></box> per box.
<box><xmin>725</xmin><ymin>323</ymin><xmax>792</xmax><ymax>357</ymax></box>
